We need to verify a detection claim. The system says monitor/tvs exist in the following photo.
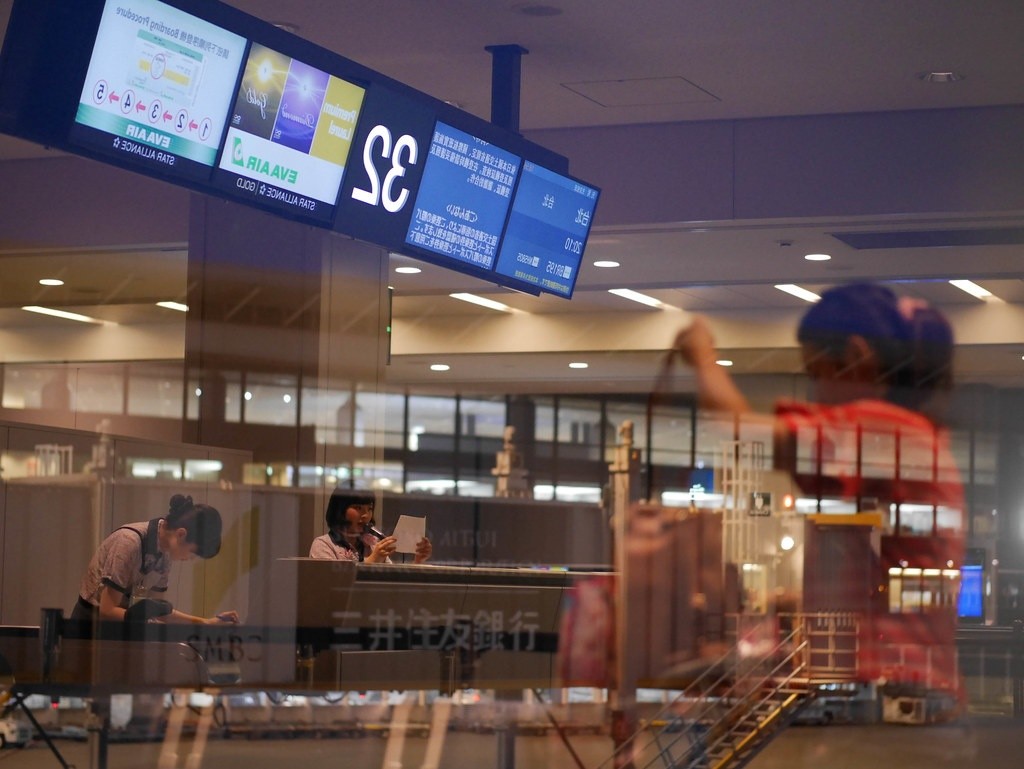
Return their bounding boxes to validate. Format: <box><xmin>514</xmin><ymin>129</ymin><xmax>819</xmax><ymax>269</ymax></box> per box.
<box><xmin>0</xmin><ymin>0</ymin><xmax>600</xmax><ymax>302</ymax></box>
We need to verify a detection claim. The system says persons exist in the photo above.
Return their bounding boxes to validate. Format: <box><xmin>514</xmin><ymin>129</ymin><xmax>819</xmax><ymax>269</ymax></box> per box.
<box><xmin>71</xmin><ymin>494</ymin><xmax>239</xmax><ymax>625</ymax></box>
<box><xmin>309</xmin><ymin>481</ymin><xmax>432</xmax><ymax>564</ymax></box>
<box><xmin>678</xmin><ymin>286</ymin><xmax>970</xmax><ymax>722</ymax></box>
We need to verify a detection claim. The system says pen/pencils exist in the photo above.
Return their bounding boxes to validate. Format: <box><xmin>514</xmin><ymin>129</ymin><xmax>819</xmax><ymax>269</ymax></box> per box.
<box><xmin>215</xmin><ymin>613</ymin><xmax>238</xmax><ymax>628</ymax></box>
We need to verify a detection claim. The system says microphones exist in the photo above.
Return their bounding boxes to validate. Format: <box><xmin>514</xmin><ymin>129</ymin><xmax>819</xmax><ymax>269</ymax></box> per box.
<box><xmin>363</xmin><ymin>521</ymin><xmax>387</xmax><ymax>541</ymax></box>
